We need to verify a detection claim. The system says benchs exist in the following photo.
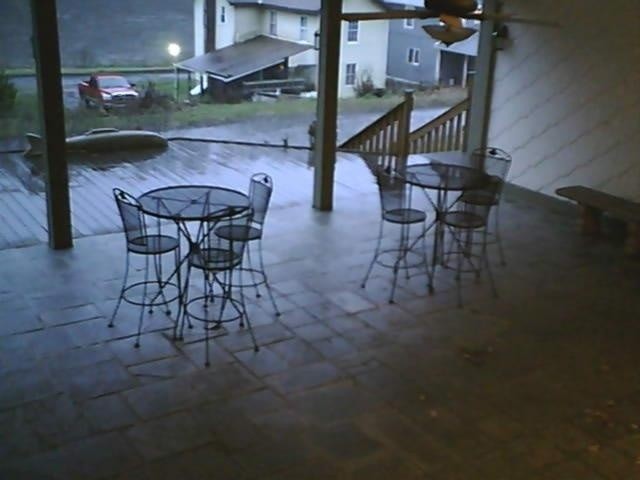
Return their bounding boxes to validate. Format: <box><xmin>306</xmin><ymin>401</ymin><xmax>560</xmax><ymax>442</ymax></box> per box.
<box><xmin>556</xmin><ymin>185</ymin><xmax>640</xmax><ymax>253</ymax></box>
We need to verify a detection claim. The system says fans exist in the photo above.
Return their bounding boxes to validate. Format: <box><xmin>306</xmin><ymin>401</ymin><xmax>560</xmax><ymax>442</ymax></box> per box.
<box><xmin>338</xmin><ymin>0</ymin><xmax>566</xmax><ymax>30</ymax></box>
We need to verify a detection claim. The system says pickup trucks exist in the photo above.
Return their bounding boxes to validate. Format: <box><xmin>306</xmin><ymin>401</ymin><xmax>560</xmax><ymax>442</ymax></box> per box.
<box><xmin>78</xmin><ymin>69</ymin><xmax>140</xmax><ymax>117</ymax></box>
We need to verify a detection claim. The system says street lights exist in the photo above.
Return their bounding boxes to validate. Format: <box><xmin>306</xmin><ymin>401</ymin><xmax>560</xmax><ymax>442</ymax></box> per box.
<box><xmin>168</xmin><ymin>44</ymin><xmax>182</xmax><ymax>102</ymax></box>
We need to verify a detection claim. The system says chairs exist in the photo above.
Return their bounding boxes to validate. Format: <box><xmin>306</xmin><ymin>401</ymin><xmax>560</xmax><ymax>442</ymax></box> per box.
<box><xmin>204</xmin><ymin>171</ymin><xmax>281</xmax><ymax>326</ymax></box>
<box><xmin>177</xmin><ymin>205</ymin><xmax>260</xmax><ymax>367</ymax></box>
<box><xmin>360</xmin><ymin>167</ymin><xmax>435</xmax><ymax>304</ymax></box>
<box><xmin>445</xmin><ymin>146</ymin><xmax>511</xmax><ymax>267</ymax></box>
<box><xmin>106</xmin><ymin>187</ymin><xmax>193</xmax><ymax>331</ymax></box>
<box><xmin>430</xmin><ymin>172</ymin><xmax>499</xmax><ymax>309</ymax></box>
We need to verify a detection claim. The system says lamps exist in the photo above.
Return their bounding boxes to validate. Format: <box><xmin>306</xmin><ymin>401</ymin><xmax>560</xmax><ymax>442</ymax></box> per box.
<box><xmin>421</xmin><ymin>15</ymin><xmax>480</xmax><ymax>47</ymax></box>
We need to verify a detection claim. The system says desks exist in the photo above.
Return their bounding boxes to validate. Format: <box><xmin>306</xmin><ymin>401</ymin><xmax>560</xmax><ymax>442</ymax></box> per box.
<box><xmin>133</xmin><ymin>185</ymin><xmax>253</xmax><ymax>342</ymax></box>
<box><xmin>396</xmin><ymin>164</ymin><xmax>492</xmax><ymax>294</ymax></box>
